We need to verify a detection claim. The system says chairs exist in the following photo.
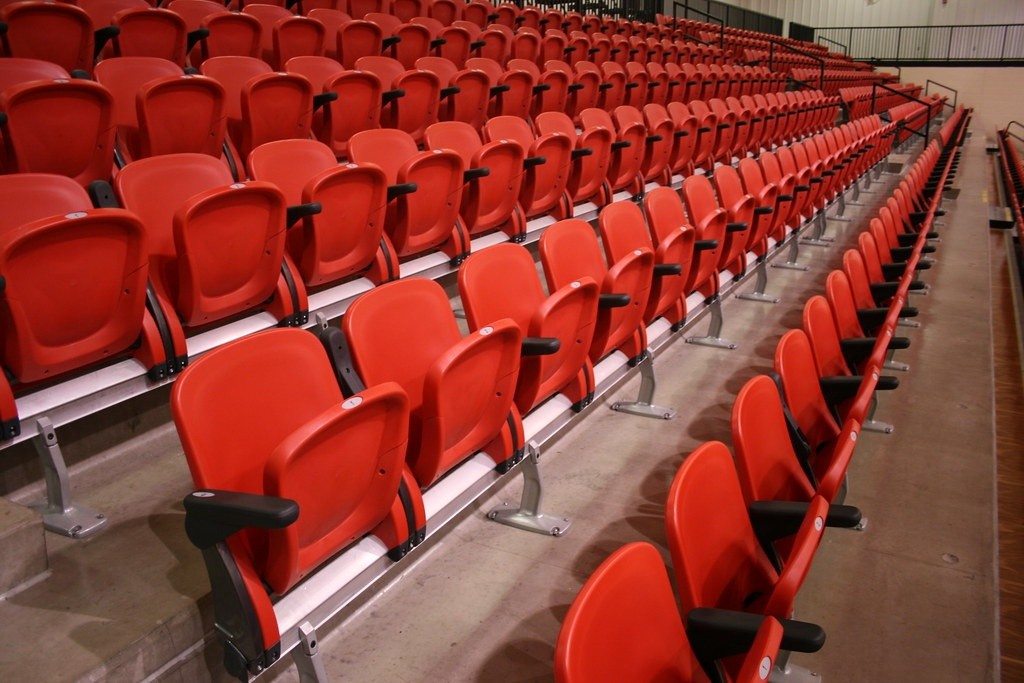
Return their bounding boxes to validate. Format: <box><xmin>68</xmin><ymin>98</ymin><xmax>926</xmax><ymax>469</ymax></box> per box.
<box><xmin>0</xmin><ymin>0</ymin><xmax>1024</xmax><ymax>683</ymax></box>
